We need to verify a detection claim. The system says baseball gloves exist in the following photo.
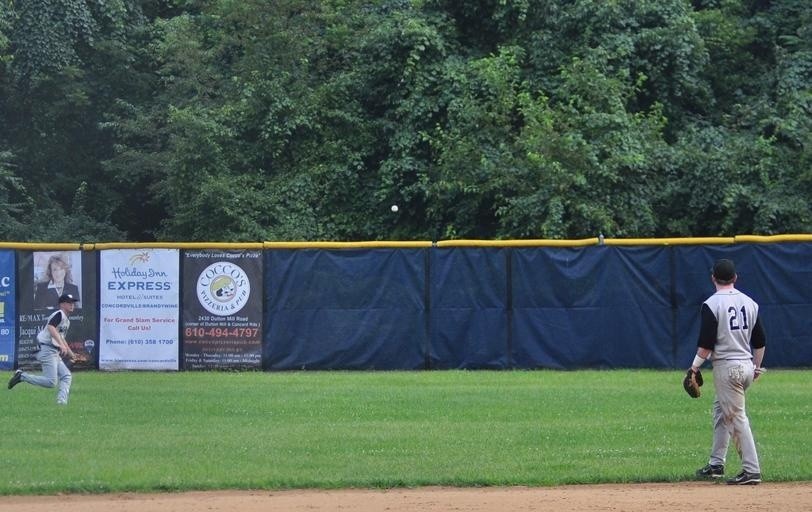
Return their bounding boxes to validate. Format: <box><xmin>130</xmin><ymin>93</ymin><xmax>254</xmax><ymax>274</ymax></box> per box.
<box><xmin>69</xmin><ymin>353</ymin><xmax>88</xmax><ymax>368</ymax></box>
<box><xmin>683</xmin><ymin>368</ymin><xmax>703</xmax><ymax>398</ymax></box>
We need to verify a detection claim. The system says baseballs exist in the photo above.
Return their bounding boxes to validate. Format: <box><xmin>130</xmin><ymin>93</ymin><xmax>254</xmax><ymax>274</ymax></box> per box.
<box><xmin>391</xmin><ymin>206</ymin><xmax>398</xmax><ymax>212</ymax></box>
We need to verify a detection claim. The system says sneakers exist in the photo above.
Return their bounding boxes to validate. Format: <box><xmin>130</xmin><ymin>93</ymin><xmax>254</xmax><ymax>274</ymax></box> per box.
<box><xmin>726</xmin><ymin>469</ymin><xmax>761</xmax><ymax>485</ymax></box>
<box><xmin>8</xmin><ymin>369</ymin><xmax>23</xmax><ymax>389</ymax></box>
<box><xmin>696</xmin><ymin>463</ymin><xmax>725</xmax><ymax>478</ymax></box>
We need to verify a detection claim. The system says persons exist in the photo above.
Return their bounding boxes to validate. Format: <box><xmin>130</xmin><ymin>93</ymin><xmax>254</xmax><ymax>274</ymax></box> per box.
<box><xmin>33</xmin><ymin>254</ymin><xmax>81</xmax><ymax>308</ymax></box>
<box><xmin>8</xmin><ymin>293</ymin><xmax>88</xmax><ymax>404</ymax></box>
<box><xmin>681</xmin><ymin>257</ymin><xmax>767</xmax><ymax>484</ymax></box>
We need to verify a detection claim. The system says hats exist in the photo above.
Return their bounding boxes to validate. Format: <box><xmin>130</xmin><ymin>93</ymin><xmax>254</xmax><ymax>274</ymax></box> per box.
<box><xmin>58</xmin><ymin>293</ymin><xmax>78</xmax><ymax>304</ymax></box>
<box><xmin>709</xmin><ymin>258</ymin><xmax>736</xmax><ymax>281</ymax></box>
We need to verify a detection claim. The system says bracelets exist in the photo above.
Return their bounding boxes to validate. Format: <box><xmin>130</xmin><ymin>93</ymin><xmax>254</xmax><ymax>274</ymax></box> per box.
<box><xmin>690</xmin><ymin>355</ymin><xmax>705</xmax><ymax>368</ymax></box>
